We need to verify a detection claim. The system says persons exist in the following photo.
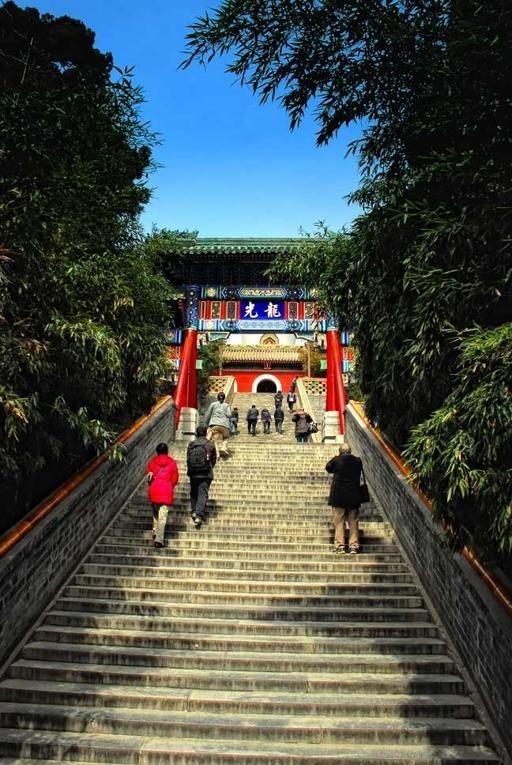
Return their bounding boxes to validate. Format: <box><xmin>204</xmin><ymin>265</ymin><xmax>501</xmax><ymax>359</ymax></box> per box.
<box><xmin>325</xmin><ymin>442</ymin><xmax>363</xmax><ymax>555</ymax></box>
<box><xmin>187</xmin><ymin>427</ymin><xmax>216</xmax><ymax>525</ymax></box>
<box><xmin>229</xmin><ymin>375</ymin><xmax>313</xmax><ymax>442</ymax></box>
<box><xmin>205</xmin><ymin>392</ymin><xmax>233</xmax><ymax>460</ymax></box>
<box><xmin>147</xmin><ymin>443</ymin><xmax>178</xmax><ymax>548</ymax></box>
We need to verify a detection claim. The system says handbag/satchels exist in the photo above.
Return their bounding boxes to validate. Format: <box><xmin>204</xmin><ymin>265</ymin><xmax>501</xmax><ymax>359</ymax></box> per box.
<box><xmin>360</xmin><ymin>483</ymin><xmax>370</xmax><ymax>504</ymax></box>
<box><xmin>309</xmin><ymin>422</ymin><xmax>318</xmax><ymax>433</ymax></box>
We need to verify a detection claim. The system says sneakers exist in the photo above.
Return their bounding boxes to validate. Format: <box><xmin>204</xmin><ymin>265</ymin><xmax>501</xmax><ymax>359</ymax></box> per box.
<box><xmin>155</xmin><ymin>542</ymin><xmax>167</xmax><ymax>548</ymax></box>
<box><xmin>194</xmin><ymin>518</ymin><xmax>205</xmax><ymax>524</ymax></box>
<box><xmin>220</xmin><ymin>449</ymin><xmax>231</xmax><ymax>457</ymax></box>
<box><xmin>348</xmin><ymin>547</ymin><xmax>358</xmax><ymax>555</ymax></box>
<box><xmin>191</xmin><ymin>512</ymin><xmax>198</xmax><ymax>521</ymax></box>
<box><xmin>332</xmin><ymin>548</ymin><xmax>346</xmax><ymax>554</ymax></box>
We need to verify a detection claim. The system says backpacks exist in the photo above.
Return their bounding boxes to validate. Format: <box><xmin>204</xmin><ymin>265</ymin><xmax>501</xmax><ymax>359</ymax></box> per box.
<box><xmin>188</xmin><ymin>440</ymin><xmax>211</xmax><ymax>473</ymax></box>
